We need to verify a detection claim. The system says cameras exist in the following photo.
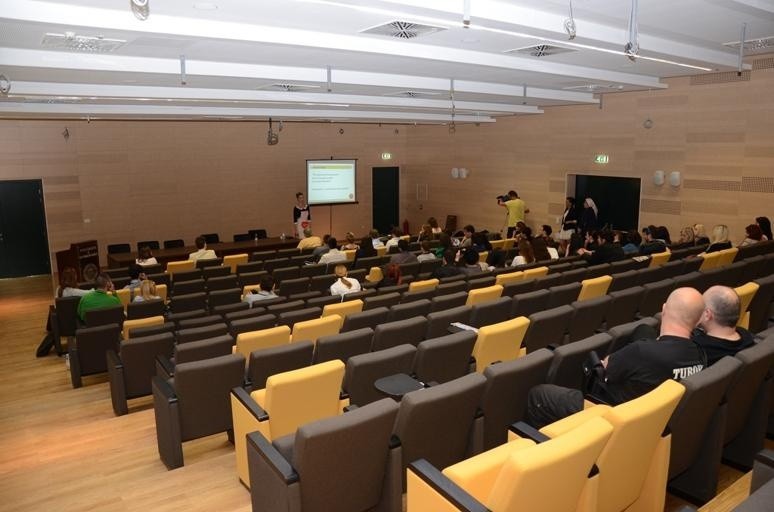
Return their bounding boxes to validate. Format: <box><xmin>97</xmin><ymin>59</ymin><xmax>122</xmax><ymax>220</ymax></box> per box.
<box><xmin>496</xmin><ymin>192</ymin><xmax>513</xmax><ymax>205</ymax></box>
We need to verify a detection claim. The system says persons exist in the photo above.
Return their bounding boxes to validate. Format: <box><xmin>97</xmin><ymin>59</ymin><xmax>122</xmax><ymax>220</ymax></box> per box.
<box><xmin>513</xmin><ymin>227</ymin><xmax>535</xmax><ymax>247</ymax></box>
<box><xmin>694</xmin><ymin>224</ymin><xmax>710</xmax><ymax>246</ymax></box>
<box><xmin>435</xmin><ymin>232</ymin><xmax>454</xmax><ymax>258</ymax></box>
<box><xmin>457</xmin><ymin>247</ymin><xmax>482</xmax><ymax>274</ymax></box>
<box><xmin>543</xmin><ymin>236</ymin><xmax>559</xmax><ymax>259</ymax></box>
<box><xmin>705</xmin><ymin>223</ymin><xmax>732</xmax><ymax>254</ymax></box>
<box><xmin>432</xmin><ymin>248</ymin><xmax>461</xmax><ymax>278</ymax></box>
<box><xmin>613</xmin><ymin>228</ymin><xmax>624</xmax><ymax>247</ymax></box>
<box><xmin>340</xmin><ymin>232</ymin><xmax>360</xmax><ymax>251</ymax></box>
<box><xmin>384</xmin><ymin>262</ymin><xmax>403</xmax><ymax>286</ymax></box>
<box><xmin>511</xmin><ymin>239</ymin><xmax>536</xmax><ymax>267</ymax></box>
<box><xmin>317</xmin><ymin>237</ymin><xmax>347</xmax><ymax>264</ymax></box>
<box><xmin>242</xmin><ymin>277</ymin><xmax>279</xmax><ymax>308</ymax></box>
<box><xmin>559</xmin><ymin>197</ymin><xmax>579</xmax><ymax>257</ymax></box>
<box><xmin>369</xmin><ymin>228</ymin><xmax>384</xmax><ymax>247</ymax></box>
<box><xmin>458</xmin><ymin>224</ymin><xmax>475</xmax><ymax>248</ymax></box>
<box><xmin>486</xmin><ymin>246</ymin><xmax>509</xmax><ymax>272</ymax></box>
<box><xmin>417</xmin><ymin>223</ymin><xmax>434</xmax><ymax>243</ymax></box>
<box><xmin>330</xmin><ymin>264</ymin><xmax>361</xmax><ymax>302</ymax></box>
<box><xmin>642</xmin><ymin>227</ymin><xmax>648</xmax><ymax>241</ymax></box>
<box><xmin>741</xmin><ymin>224</ymin><xmax>762</xmax><ymax>247</ymax></box>
<box><xmin>755</xmin><ymin>216</ymin><xmax>773</xmax><ymax>241</ymax></box>
<box><xmin>534</xmin><ymin>225</ymin><xmax>556</xmax><ymax>247</ymax></box>
<box><xmin>132</xmin><ymin>279</ymin><xmax>161</xmax><ymax>302</ymax></box>
<box><xmin>297</xmin><ymin>228</ymin><xmax>322</xmax><ymax>248</ymax></box>
<box><xmin>426</xmin><ymin>216</ymin><xmax>442</xmax><ymax>233</ymax></box>
<box><xmin>526</xmin><ymin>287</ymin><xmax>707</xmax><ymax>429</ymax></box>
<box><xmin>496</xmin><ymin>190</ymin><xmax>530</xmax><ymax>238</ymax></box>
<box><xmin>312</xmin><ymin>234</ymin><xmax>331</xmax><ymax>256</ymax></box>
<box><xmin>645</xmin><ymin>225</ymin><xmax>656</xmax><ymax>242</ymax></box>
<box><xmin>576</xmin><ymin>198</ymin><xmax>602</xmax><ymax>234</ymax></box>
<box><xmin>392</xmin><ymin>239</ymin><xmax>418</xmax><ymax>264</ymax></box>
<box><xmin>76</xmin><ymin>272</ymin><xmax>122</xmax><ymax>327</ymax></box>
<box><xmin>360</xmin><ymin>267</ymin><xmax>383</xmax><ymax>287</ymax></box>
<box><xmin>354</xmin><ymin>236</ymin><xmax>377</xmax><ymax>261</ymax></box>
<box><xmin>512</xmin><ymin>221</ymin><xmax>526</xmax><ymax>238</ymax></box>
<box><xmin>583</xmin><ymin>228</ymin><xmax>598</xmax><ymax>251</ymax></box>
<box><xmin>54</xmin><ymin>264</ymin><xmax>95</xmax><ymax>309</ymax></box>
<box><xmin>470</xmin><ymin>232</ymin><xmax>493</xmax><ymax>252</ymax></box>
<box><xmin>386</xmin><ymin>227</ymin><xmax>402</xmax><ymax>253</ymax></box>
<box><xmin>632</xmin><ymin>284</ymin><xmax>756</xmax><ymax>367</ymax></box>
<box><xmin>124</xmin><ymin>264</ymin><xmax>149</xmax><ymax>289</ymax></box>
<box><xmin>678</xmin><ymin>224</ymin><xmax>695</xmax><ymax>249</ymax></box>
<box><xmin>135</xmin><ymin>246</ymin><xmax>158</xmax><ymax>266</ymax></box>
<box><xmin>639</xmin><ymin>240</ymin><xmax>667</xmax><ymax>256</ymax></box>
<box><xmin>188</xmin><ymin>237</ymin><xmax>217</xmax><ymax>268</ymax></box>
<box><xmin>417</xmin><ymin>240</ymin><xmax>436</xmax><ymax>263</ymax></box>
<box><xmin>656</xmin><ymin>226</ymin><xmax>672</xmax><ymax>245</ymax></box>
<box><xmin>293</xmin><ymin>192</ymin><xmax>311</xmax><ymax>239</ymax></box>
<box><xmin>527</xmin><ymin>237</ymin><xmax>551</xmax><ymax>262</ymax></box>
<box><xmin>577</xmin><ymin>229</ymin><xmax>625</xmax><ymax>265</ymax></box>
<box><xmin>622</xmin><ymin>228</ymin><xmax>643</xmax><ymax>252</ymax></box>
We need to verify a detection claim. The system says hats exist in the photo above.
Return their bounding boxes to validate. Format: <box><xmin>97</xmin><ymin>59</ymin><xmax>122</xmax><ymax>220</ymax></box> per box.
<box><xmin>585</xmin><ymin>197</ymin><xmax>598</xmax><ymax>216</ymax></box>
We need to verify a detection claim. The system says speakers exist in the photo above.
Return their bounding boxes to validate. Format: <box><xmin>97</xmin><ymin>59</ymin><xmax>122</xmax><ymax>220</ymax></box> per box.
<box><xmin>55</xmin><ymin>252</ymin><xmax>80</xmax><ymax>295</ymax></box>
<box><xmin>69</xmin><ymin>240</ymin><xmax>98</xmax><ymax>290</ymax></box>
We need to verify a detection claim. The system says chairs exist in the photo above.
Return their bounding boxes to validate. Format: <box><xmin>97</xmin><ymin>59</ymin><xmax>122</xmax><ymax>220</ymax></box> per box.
<box><xmin>47</xmin><ymin>229</ymin><xmax>774</xmax><ymax>512</ymax></box>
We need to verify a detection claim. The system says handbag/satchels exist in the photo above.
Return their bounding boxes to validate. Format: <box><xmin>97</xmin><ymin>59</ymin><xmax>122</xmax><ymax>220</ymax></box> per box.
<box><xmin>581</xmin><ymin>350</ymin><xmax>606</xmax><ymax>396</ymax></box>
